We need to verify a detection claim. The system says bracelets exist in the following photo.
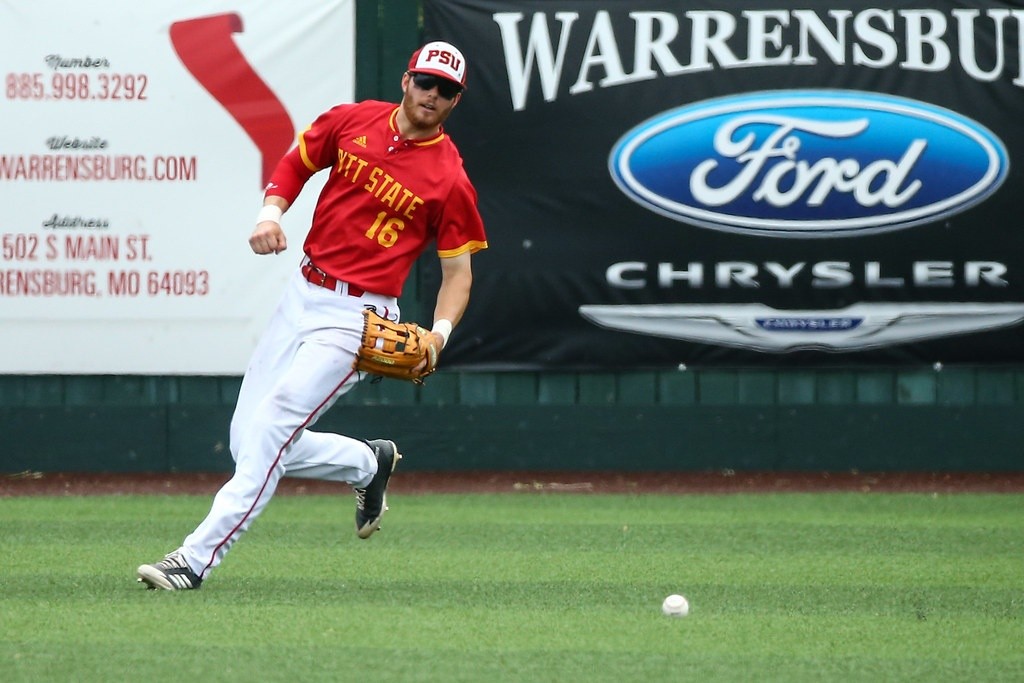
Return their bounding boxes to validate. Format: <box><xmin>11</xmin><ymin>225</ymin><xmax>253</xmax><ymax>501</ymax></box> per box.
<box><xmin>430</xmin><ymin>318</ymin><xmax>452</xmax><ymax>351</ymax></box>
<box><xmin>255</xmin><ymin>205</ymin><xmax>283</xmax><ymax>228</ymax></box>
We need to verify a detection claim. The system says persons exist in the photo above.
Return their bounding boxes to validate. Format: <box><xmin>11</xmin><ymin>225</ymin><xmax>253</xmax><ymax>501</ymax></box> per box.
<box><xmin>136</xmin><ymin>40</ymin><xmax>488</xmax><ymax>591</ymax></box>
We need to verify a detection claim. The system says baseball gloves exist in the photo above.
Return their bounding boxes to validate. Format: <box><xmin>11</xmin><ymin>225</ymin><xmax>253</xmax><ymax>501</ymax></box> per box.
<box><xmin>351</xmin><ymin>304</ymin><xmax>440</xmax><ymax>386</ymax></box>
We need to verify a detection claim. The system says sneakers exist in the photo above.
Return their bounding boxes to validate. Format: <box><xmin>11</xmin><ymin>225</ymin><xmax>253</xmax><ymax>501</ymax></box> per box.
<box><xmin>138</xmin><ymin>553</ymin><xmax>203</xmax><ymax>592</ymax></box>
<box><xmin>354</xmin><ymin>438</ymin><xmax>402</xmax><ymax>539</ymax></box>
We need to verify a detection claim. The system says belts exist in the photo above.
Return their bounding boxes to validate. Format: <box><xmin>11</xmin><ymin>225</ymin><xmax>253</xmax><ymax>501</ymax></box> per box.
<box><xmin>302</xmin><ymin>265</ymin><xmax>365</xmax><ymax>297</ymax></box>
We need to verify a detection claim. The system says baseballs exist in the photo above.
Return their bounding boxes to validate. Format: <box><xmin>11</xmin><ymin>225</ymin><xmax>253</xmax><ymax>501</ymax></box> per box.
<box><xmin>662</xmin><ymin>594</ymin><xmax>689</xmax><ymax>616</ymax></box>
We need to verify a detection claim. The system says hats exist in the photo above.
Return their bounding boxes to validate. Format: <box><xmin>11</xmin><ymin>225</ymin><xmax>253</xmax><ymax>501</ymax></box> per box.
<box><xmin>408</xmin><ymin>42</ymin><xmax>467</xmax><ymax>87</ymax></box>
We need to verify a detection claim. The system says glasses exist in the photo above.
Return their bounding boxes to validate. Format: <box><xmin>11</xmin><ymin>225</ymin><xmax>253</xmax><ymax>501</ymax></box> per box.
<box><xmin>407</xmin><ymin>70</ymin><xmax>462</xmax><ymax>100</ymax></box>
